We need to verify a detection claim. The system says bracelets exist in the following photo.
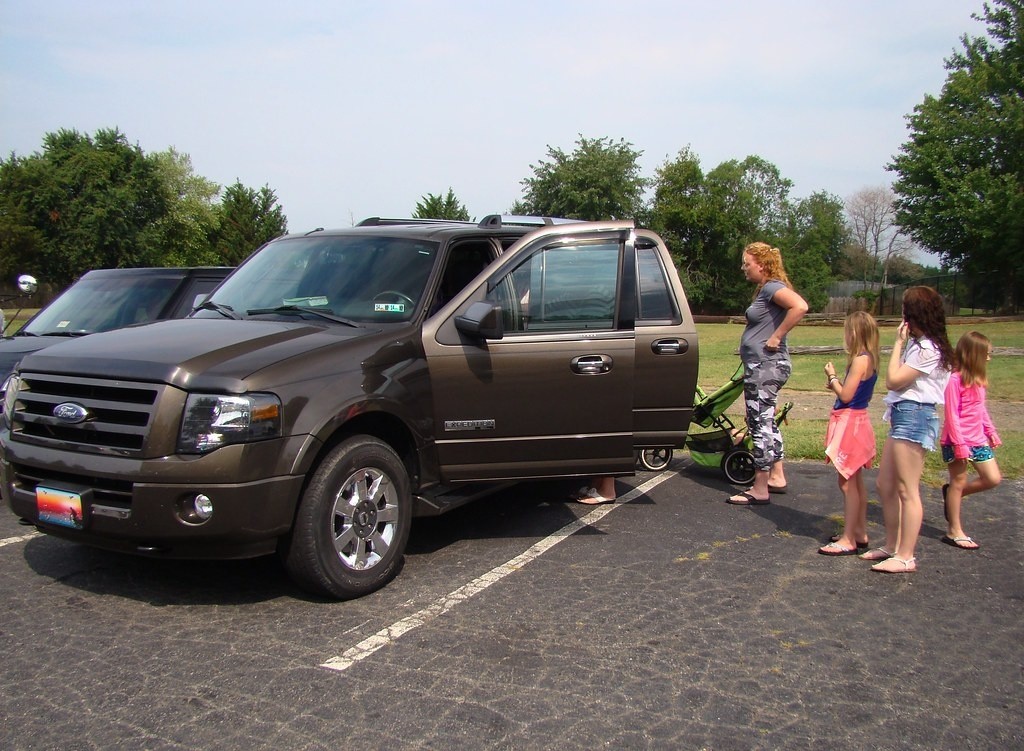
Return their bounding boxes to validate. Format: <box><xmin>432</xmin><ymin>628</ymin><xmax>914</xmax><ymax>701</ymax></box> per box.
<box><xmin>828</xmin><ymin>374</ymin><xmax>840</xmax><ymax>384</ymax></box>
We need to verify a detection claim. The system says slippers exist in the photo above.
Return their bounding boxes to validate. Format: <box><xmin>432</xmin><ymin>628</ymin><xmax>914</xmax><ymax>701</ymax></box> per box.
<box><xmin>828</xmin><ymin>534</ymin><xmax>868</xmax><ymax>548</ymax></box>
<box><xmin>576</xmin><ymin>488</ymin><xmax>616</xmax><ymax>504</ymax></box>
<box><xmin>942</xmin><ymin>483</ymin><xmax>949</xmax><ymax>522</ymax></box>
<box><xmin>818</xmin><ymin>542</ymin><xmax>858</xmax><ymax>556</ymax></box>
<box><xmin>725</xmin><ymin>489</ymin><xmax>771</xmax><ymax>505</ymax></box>
<box><xmin>942</xmin><ymin>534</ymin><xmax>980</xmax><ymax>549</ymax></box>
<box><xmin>568</xmin><ymin>486</ymin><xmax>592</xmax><ymax>499</ymax></box>
<box><xmin>746</xmin><ymin>481</ymin><xmax>789</xmax><ymax>493</ymax></box>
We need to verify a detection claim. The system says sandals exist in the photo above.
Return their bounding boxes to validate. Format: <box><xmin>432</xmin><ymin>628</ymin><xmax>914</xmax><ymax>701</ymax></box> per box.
<box><xmin>870</xmin><ymin>557</ymin><xmax>917</xmax><ymax>573</ymax></box>
<box><xmin>858</xmin><ymin>546</ymin><xmax>898</xmax><ymax>560</ymax></box>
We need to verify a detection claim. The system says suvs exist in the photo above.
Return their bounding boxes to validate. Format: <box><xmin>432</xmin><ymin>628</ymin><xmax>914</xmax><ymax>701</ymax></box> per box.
<box><xmin>0</xmin><ymin>215</ymin><xmax>700</xmax><ymax>600</ymax></box>
<box><xmin>1</xmin><ymin>266</ymin><xmax>240</xmax><ymax>405</ymax></box>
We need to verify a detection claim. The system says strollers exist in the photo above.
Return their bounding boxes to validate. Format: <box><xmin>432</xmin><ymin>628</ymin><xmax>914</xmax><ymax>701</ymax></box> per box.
<box><xmin>639</xmin><ymin>360</ymin><xmax>794</xmax><ymax>485</ymax></box>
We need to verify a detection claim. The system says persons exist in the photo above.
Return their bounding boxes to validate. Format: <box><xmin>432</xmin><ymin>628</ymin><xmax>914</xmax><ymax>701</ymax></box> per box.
<box><xmin>818</xmin><ymin>312</ymin><xmax>879</xmax><ymax>556</ymax></box>
<box><xmin>726</xmin><ymin>242</ymin><xmax>808</xmax><ymax>505</ymax></box>
<box><xmin>858</xmin><ymin>286</ymin><xmax>959</xmax><ymax>572</ymax></box>
<box><xmin>544</xmin><ymin>258</ymin><xmax>616</xmax><ymax>505</ymax></box>
<box><xmin>942</xmin><ymin>330</ymin><xmax>1001</xmax><ymax>551</ymax></box>
